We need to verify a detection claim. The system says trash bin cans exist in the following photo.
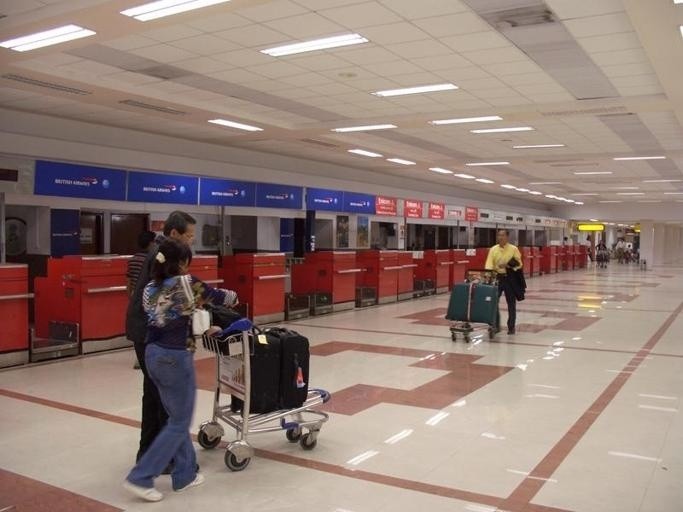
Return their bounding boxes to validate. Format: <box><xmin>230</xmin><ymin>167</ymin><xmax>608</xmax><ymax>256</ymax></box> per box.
<box><xmin>641</xmin><ymin>260</ymin><xmax>646</xmax><ymax>271</ymax></box>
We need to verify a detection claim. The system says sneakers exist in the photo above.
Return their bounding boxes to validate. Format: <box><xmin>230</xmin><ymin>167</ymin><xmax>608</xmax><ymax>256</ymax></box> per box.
<box><xmin>174</xmin><ymin>471</ymin><xmax>205</xmax><ymax>493</ymax></box>
<box><xmin>123</xmin><ymin>478</ymin><xmax>161</xmax><ymax>502</ymax></box>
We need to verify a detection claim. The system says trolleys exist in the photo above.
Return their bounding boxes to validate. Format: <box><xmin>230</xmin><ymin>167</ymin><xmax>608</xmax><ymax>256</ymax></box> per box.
<box><xmin>447</xmin><ymin>267</ymin><xmax>503</xmax><ymax>341</ymax></box>
<box><xmin>195</xmin><ymin>296</ymin><xmax>335</xmax><ymax>474</ymax></box>
<box><xmin>590</xmin><ymin>248</ymin><xmax>642</xmax><ymax>269</ymax></box>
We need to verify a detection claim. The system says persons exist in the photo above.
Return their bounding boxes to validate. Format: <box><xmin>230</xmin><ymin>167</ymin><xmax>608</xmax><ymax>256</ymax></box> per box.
<box><xmin>595</xmin><ymin>239</ymin><xmax>607</xmax><ymax>268</ymax></box>
<box><xmin>122</xmin><ymin>211</ymin><xmax>239</xmax><ymax>502</ymax></box>
<box><xmin>484</xmin><ymin>229</ymin><xmax>523</xmax><ymax>335</ymax></box>
<box><xmin>563</xmin><ymin>236</ymin><xmax>568</xmax><ymax>245</ymax></box>
<box><xmin>615</xmin><ymin>239</ymin><xmax>638</xmax><ymax>264</ymax></box>
<box><xmin>585</xmin><ymin>237</ymin><xmax>594</xmax><ymax>261</ymax></box>
<box><xmin>126</xmin><ymin>231</ymin><xmax>156</xmax><ymax>370</ymax></box>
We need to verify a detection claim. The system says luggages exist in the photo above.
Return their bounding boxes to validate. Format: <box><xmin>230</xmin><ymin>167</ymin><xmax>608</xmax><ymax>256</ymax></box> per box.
<box><xmin>445</xmin><ymin>283</ymin><xmax>499</xmax><ymax>325</ymax></box>
<box><xmin>508</xmin><ymin>326</ymin><xmax>514</xmax><ymax>334</ymax></box>
<box><xmin>231</xmin><ymin>327</ymin><xmax>309</xmax><ymax>413</ymax></box>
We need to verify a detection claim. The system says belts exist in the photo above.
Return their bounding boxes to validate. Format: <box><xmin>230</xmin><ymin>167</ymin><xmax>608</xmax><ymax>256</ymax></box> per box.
<box><xmin>498</xmin><ymin>274</ymin><xmax>511</xmax><ymax>276</ymax></box>
